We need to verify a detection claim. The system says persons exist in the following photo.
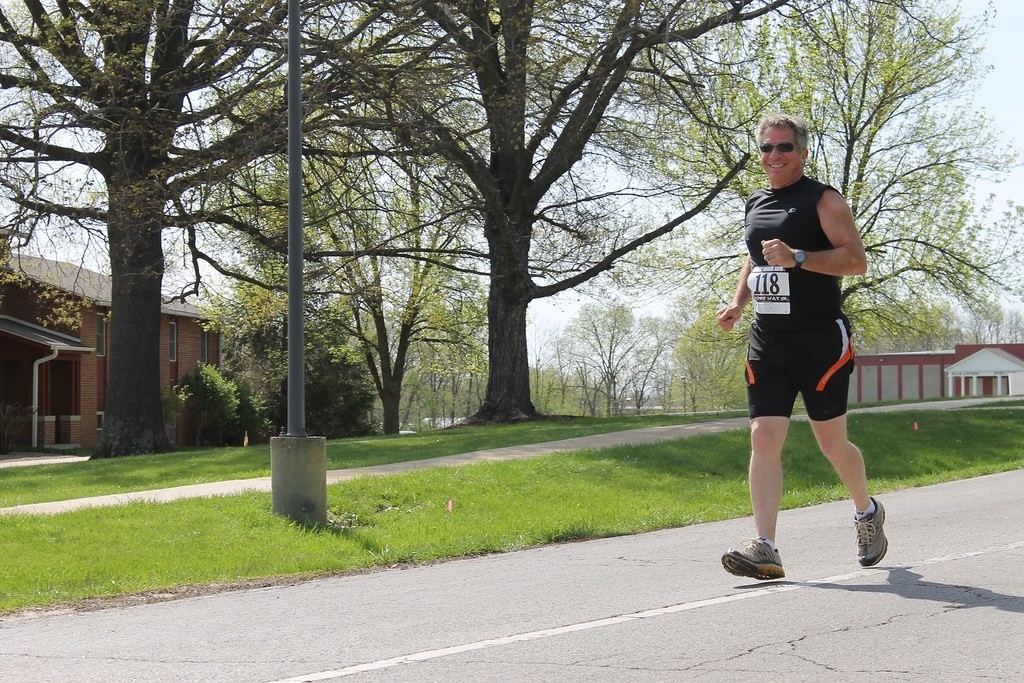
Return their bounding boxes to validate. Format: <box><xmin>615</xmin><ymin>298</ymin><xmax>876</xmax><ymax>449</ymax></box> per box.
<box><xmin>716</xmin><ymin>115</ymin><xmax>887</xmax><ymax>581</ymax></box>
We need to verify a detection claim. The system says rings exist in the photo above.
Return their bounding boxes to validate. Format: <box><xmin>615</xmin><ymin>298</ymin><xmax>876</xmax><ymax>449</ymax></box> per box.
<box><xmin>767</xmin><ymin>254</ymin><xmax>771</xmax><ymax>260</ymax></box>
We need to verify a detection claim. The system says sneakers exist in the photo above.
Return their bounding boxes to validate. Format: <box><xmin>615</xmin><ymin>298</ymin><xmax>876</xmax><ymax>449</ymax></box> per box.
<box><xmin>854</xmin><ymin>497</ymin><xmax>888</xmax><ymax>566</ymax></box>
<box><xmin>721</xmin><ymin>539</ymin><xmax>786</xmax><ymax>580</ymax></box>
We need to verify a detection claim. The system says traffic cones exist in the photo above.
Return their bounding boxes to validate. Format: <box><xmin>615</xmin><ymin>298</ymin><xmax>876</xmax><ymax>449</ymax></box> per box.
<box><xmin>913</xmin><ymin>422</ymin><xmax>919</xmax><ymax>431</ymax></box>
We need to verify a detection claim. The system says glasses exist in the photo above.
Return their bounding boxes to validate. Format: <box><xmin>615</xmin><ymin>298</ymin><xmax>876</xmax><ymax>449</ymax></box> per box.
<box><xmin>758</xmin><ymin>141</ymin><xmax>804</xmax><ymax>153</ymax></box>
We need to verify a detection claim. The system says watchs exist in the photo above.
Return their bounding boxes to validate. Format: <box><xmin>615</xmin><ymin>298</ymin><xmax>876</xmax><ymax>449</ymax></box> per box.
<box><xmin>793</xmin><ymin>249</ymin><xmax>805</xmax><ymax>268</ymax></box>
